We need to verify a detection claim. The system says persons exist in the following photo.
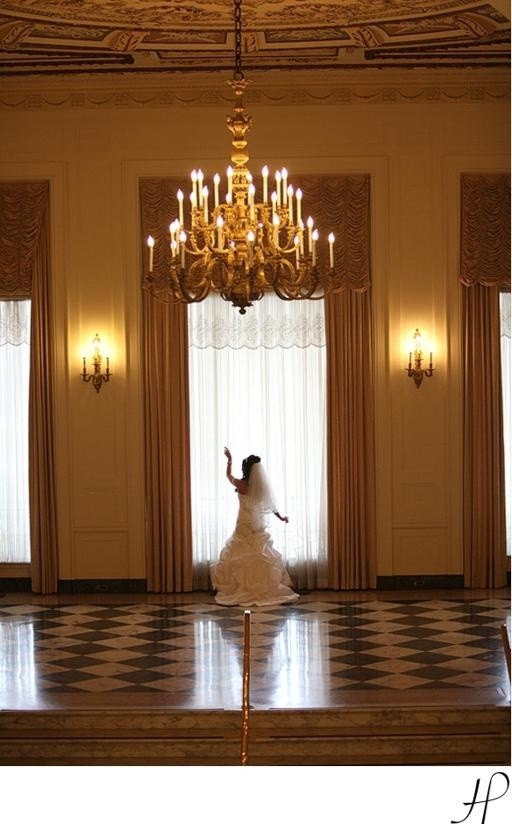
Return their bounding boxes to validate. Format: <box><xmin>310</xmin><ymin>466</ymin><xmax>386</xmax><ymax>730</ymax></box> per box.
<box><xmin>211</xmin><ymin>447</ymin><xmax>301</xmax><ymax>606</ymax></box>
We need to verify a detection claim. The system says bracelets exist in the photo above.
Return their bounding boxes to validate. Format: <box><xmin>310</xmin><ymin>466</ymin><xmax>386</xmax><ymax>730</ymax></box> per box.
<box><xmin>227</xmin><ymin>460</ymin><xmax>233</xmax><ymax>465</ymax></box>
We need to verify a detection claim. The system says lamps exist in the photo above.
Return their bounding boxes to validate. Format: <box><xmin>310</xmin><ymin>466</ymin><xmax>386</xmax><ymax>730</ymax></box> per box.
<box><xmin>403</xmin><ymin>327</ymin><xmax>435</xmax><ymax>391</ymax></box>
<box><xmin>140</xmin><ymin>0</ymin><xmax>339</xmax><ymax>317</ymax></box>
<box><xmin>78</xmin><ymin>331</ymin><xmax>114</xmax><ymax>395</ymax></box>
